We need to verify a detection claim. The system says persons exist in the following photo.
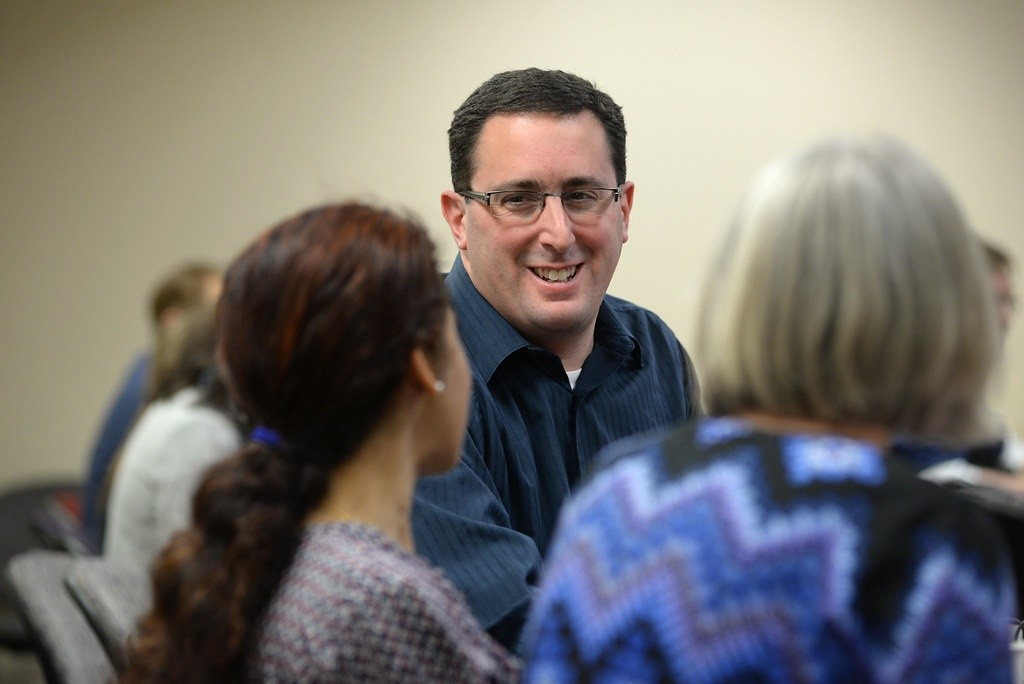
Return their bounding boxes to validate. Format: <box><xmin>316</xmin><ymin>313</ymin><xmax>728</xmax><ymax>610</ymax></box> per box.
<box><xmin>887</xmin><ymin>244</ymin><xmax>1023</xmax><ymax>497</ymax></box>
<box><xmin>83</xmin><ymin>203</ymin><xmax>527</xmax><ymax>684</ymax></box>
<box><xmin>521</xmin><ymin>147</ymin><xmax>1023</xmax><ymax>683</ymax></box>
<box><xmin>408</xmin><ymin>67</ymin><xmax>701</xmax><ymax>648</ymax></box>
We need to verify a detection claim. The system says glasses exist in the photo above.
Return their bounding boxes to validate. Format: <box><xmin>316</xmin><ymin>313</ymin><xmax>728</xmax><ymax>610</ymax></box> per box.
<box><xmin>460</xmin><ymin>187</ymin><xmax>621</xmax><ymax>225</ymax></box>
